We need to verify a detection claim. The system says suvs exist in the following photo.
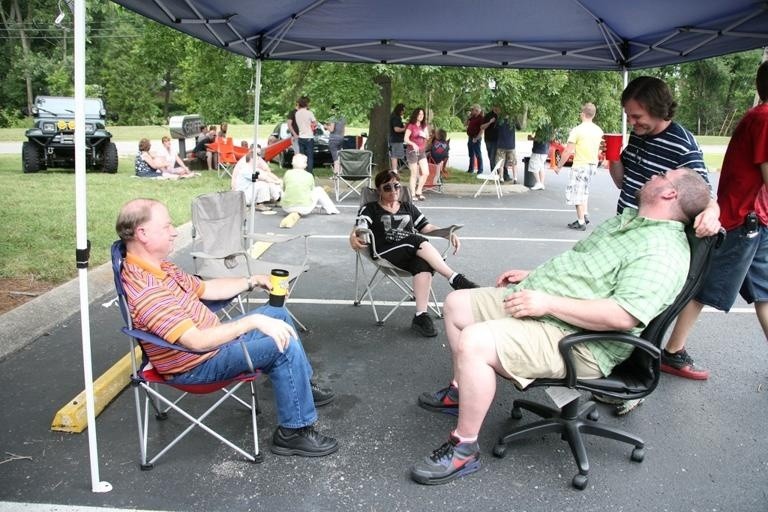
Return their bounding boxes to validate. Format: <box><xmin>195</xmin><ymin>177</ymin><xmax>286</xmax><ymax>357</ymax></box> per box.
<box><xmin>23</xmin><ymin>95</ymin><xmax>120</xmax><ymax>176</ymax></box>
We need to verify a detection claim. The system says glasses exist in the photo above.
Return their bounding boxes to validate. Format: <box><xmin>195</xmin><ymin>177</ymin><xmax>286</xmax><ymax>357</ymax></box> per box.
<box><xmin>383</xmin><ymin>183</ymin><xmax>401</xmax><ymax>192</ymax></box>
<box><xmin>658</xmin><ymin>171</ymin><xmax>676</xmax><ymax>189</ymax></box>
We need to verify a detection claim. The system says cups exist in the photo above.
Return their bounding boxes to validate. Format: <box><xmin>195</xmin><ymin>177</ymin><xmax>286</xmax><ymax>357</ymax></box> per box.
<box><xmin>602</xmin><ymin>133</ymin><xmax>624</xmax><ymax>163</ymax></box>
<box><xmin>268</xmin><ymin>269</ymin><xmax>290</xmax><ymax>308</ymax></box>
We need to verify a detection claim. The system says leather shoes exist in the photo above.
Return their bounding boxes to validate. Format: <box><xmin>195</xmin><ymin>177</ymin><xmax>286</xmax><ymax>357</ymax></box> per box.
<box><xmin>310</xmin><ymin>379</ymin><xmax>334</xmax><ymax>407</ymax></box>
<box><xmin>271</xmin><ymin>426</ymin><xmax>338</xmax><ymax>457</ymax></box>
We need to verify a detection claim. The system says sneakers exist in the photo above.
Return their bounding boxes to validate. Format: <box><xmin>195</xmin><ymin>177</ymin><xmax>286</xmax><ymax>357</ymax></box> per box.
<box><xmin>531</xmin><ymin>182</ymin><xmax>545</xmax><ymax>190</ymax></box>
<box><xmin>418</xmin><ymin>380</ymin><xmax>459</xmax><ymax>417</ymax></box>
<box><xmin>593</xmin><ymin>392</ymin><xmax>645</xmax><ymax>415</ymax></box>
<box><xmin>411</xmin><ymin>431</ymin><xmax>482</xmax><ymax>485</ymax></box>
<box><xmin>660</xmin><ymin>346</ymin><xmax>709</xmax><ymax>380</ymax></box>
<box><xmin>412</xmin><ymin>312</ymin><xmax>438</xmax><ymax>337</ymax></box>
<box><xmin>568</xmin><ymin>214</ymin><xmax>589</xmax><ymax>231</ymax></box>
<box><xmin>450</xmin><ymin>273</ymin><xmax>479</xmax><ymax>290</ymax></box>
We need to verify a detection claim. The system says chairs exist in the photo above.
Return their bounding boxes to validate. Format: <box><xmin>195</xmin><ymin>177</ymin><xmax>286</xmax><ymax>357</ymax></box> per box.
<box><xmin>216</xmin><ymin>136</ymin><xmax>237</xmax><ymax>178</ymax></box>
<box><xmin>110</xmin><ymin>239</ymin><xmax>263</xmax><ymax>470</ymax></box>
<box><xmin>474</xmin><ymin>159</ymin><xmax>503</xmax><ymax>199</ymax></box>
<box><xmin>416</xmin><ymin>161</ymin><xmax>443</xmax><ymax>194</ymax></box>
<box><xmin>331</xmin><ymin>148</ymin><xmax>376</xmax><ymax>203</ymax></box>
<box><xmin>187</xmin><ymin>190</ymin><xmax>308</xmax><ymax>331</ymax></box>
<box><xmin>491</xmin><ymin>224</ymin><xmax>726</xmax><ymax>490</ymax></box>
<box><xmin>354</xmin><ymin>186</ymin><xmax>462</xmax><ymax>326</ymax></box>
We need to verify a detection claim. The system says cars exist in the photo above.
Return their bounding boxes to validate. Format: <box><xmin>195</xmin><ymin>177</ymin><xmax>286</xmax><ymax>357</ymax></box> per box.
<box><xmin>268</xmin><ymin>120</ymin><xmax>334</xmax><ymax>169</ymax></box>
<box><xmin>529</xmin><ymin>130</ymin><xmax>603</xmax><ymax>171</ymax></box>
<box><xmin>359</xmin><ymin>131</ymin><xmax>431</xmax><ymax>165</ymax></box>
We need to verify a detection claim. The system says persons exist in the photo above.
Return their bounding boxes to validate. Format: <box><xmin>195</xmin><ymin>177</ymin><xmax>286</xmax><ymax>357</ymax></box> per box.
<box><xmin>193</xmin><ymin>95</ymin><xmax>345</xmax><ymax>216</ymax></box>
<box><xmin>154</xmin><ymin>136</ymin><xmax>191</xmax><ymax>175</ymax></box>
<box><xmin>412</xmin><ymin>168</ymin><xmax>711</xmax><ymax>484</ymax></box>
<box><xmin>389</xmin><ymin>104</ymin><xmax>450</xmax><ymax>202</ymax></box>
<box><xmin>593</xmin><ymin>77</ymin><xmax>723</xmax><ymax>415</ymax></box>
<box><xmin>116</xmin><ymin>198</ymin><xmax>341</xmax><ymax>457</ymax></box>
<box><xmin>350</xmin><ymin>169</ymin><xmax>480</xmax><ymax>338</ymax></box>
<box><xmin>660</xmin><ymin>60</ymin><xmax>768</xmax><ymax>381</ymax></box>
<box><xmin>135</xmin><ymin>138</ymin><xmax>173</xmax><ymax>177</ymax></box>
<box><xmin>465</xmin><ymin>104</ymin><xmax>518</xmax><ymax>183</ymax></box>
<box><xmin>528</xmin><ymin>124</ymin><xmax>550</xmax><ymax>190</ymax></box>
<box><xmin>555</xmin><ymin>103</ymin><xmax>604</xmax><ymax>231</ymax></box>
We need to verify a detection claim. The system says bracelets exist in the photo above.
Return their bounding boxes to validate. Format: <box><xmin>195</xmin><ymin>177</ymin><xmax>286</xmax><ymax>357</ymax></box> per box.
<box><xmin>247</xmin><ymin>276</ymin><xmax>253</xmax><ymax>290</ymax></box>
<box><xmin>558</xmin><ymin>161</ymin><xmax>563</xmax><ymax>168</ymax></box>
